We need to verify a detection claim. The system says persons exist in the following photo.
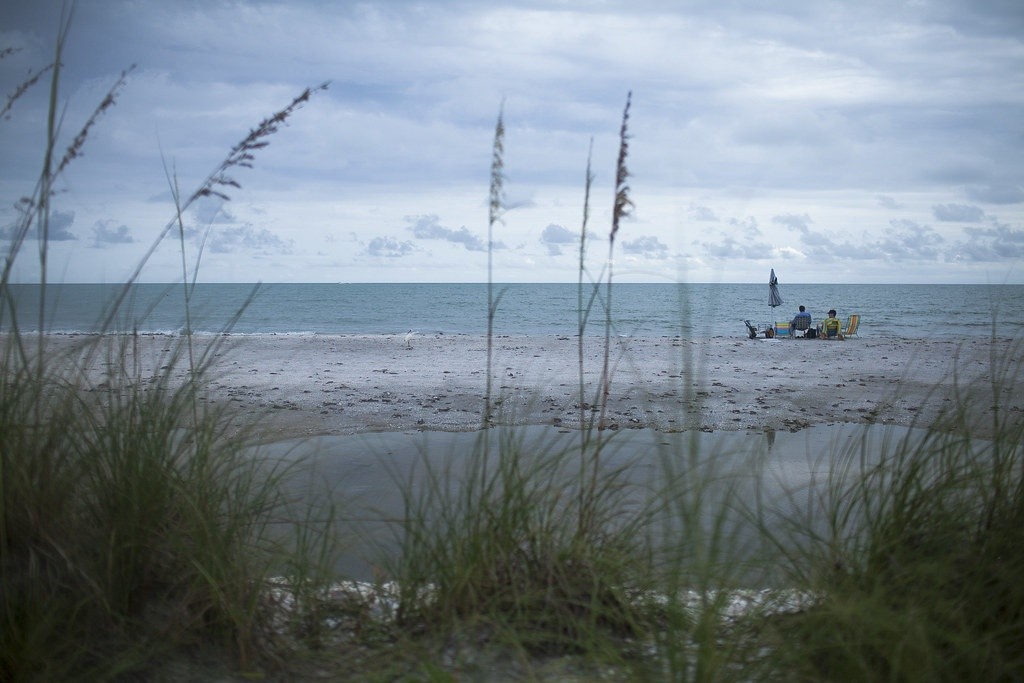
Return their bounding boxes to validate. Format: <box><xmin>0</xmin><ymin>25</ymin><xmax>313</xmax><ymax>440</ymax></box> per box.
<box><xmin>817</xmin><ymin>309</ymin><xmax>836</xmax><ymax>337</ymax></box>
<box><xmin>789</xmin><ymin>306</ymin><xmax>811</xmax><ymax>336</ymax></box>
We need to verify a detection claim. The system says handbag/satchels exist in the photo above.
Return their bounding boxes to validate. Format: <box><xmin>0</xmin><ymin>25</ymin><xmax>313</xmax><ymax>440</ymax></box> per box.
<box><xmin>806</xmin><ymin>328</ymin><xmax>816</xmax><ymax>339</ymax></box>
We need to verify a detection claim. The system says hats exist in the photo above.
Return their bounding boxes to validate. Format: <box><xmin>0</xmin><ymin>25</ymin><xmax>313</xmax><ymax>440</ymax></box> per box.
<box><xmin>827</xmin><ymin>310</ymin><xmax>836</xmax><ymax>315</ymax></box>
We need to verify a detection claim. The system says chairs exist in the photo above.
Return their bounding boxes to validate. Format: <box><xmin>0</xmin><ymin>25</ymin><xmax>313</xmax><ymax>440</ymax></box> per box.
<box><xmin>820</xmin><ymin>320</ymin><xmax>838</xmax><ymax>339</ymax></box>
<box><xmin>841</xmin><ymin>315</ymin><xmax>861</xmax><ymax>338</ymax></box>
<box><xmin>775</xmin><ymin>316</ymin><xmax>811</xmax><ymax>340</ymax></box>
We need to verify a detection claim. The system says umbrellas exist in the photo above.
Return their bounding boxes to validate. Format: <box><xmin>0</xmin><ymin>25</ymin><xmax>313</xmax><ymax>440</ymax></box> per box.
<box><xmin>768</xmin><ymin>268</ymin><xmax>784</xmax><ymax>327</ymax></box>
